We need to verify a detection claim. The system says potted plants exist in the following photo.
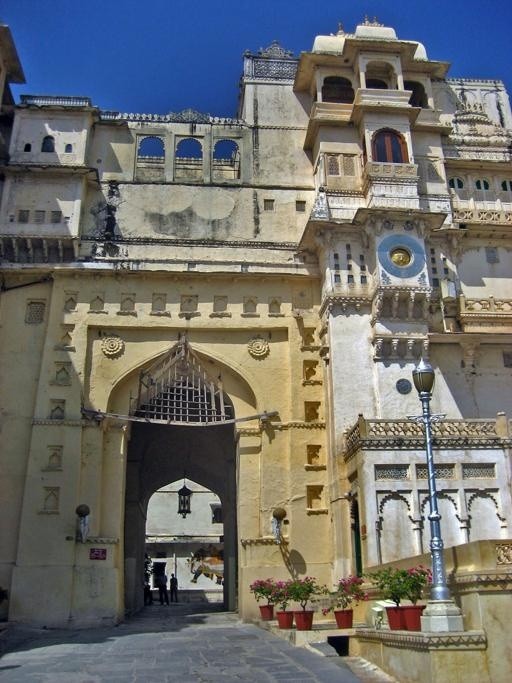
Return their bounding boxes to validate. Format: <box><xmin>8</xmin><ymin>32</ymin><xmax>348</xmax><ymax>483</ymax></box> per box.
<box><xmin>366</xmin><ymin>567</ymin><xmax>404</xmax><ymax>630</ymax></box>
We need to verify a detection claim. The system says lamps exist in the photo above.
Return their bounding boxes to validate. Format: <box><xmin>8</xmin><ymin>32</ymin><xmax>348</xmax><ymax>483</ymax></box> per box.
<box><xmin>260</xmin><ymin>411</ymin><xmax>270</xmax><ymax>428</ymax></box>
<box><xmin>272</xmin><ymin>508</ymin><xmax>286</xmax><ymax>544</ymax></box>
<box><xmin>178</xmin><ymin>470</ymin><xmax>192</xmax><ymax>519</ymax></box>
<box><xmin>94</xmin><ymin>410</ymin><xmax>107</xmax><ymax>426</ymax></box>
<box><xmin>76</xmin><ymin>504</ymin><xmax>90</xmax><ymax>544</ymax></box>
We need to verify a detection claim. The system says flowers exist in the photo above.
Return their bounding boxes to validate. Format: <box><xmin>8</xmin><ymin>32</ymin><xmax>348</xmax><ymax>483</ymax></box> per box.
<box><xmin>402</xmin><ymin>564</ymin><xmax>434</xmax><ymax>605</ymax></box>
<box><xmin>321</xmin><ymin>576</ymin><xmax>368</xmax><ymax>615</ymax></box>
<box><xmin>250</xmin><ymin>577</ymin><xmax>328</xmax><ymax>611</ymax></box>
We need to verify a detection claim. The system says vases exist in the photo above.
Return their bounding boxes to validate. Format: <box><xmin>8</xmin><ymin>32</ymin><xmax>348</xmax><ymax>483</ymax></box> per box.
<box><xmin>258</xmin><ymin>605</ymin><xmax>314</xmax><ymax>631</ymax></box>
<box><xmin>334</xmin><ymin>610</ymin><xmax>353</xmax><ymax>628</ymax></box>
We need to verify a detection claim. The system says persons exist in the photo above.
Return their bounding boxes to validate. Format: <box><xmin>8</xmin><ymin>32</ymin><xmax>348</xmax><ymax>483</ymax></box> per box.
<box><xmin>158</xmin><ymin>571</ymin><xmax>170</xmax><ymax>607</ymax></box>
<box><xmin>170</xmin><ymin>572</ymin><xmax>178</xmax><ymax>602</ymax></box>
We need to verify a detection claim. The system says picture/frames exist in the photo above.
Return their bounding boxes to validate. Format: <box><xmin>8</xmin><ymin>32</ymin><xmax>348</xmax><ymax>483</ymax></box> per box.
<box><xmin>400</xmin><ymin>605</ymin><xmax>426</xmax><ymax>631</ymax></box>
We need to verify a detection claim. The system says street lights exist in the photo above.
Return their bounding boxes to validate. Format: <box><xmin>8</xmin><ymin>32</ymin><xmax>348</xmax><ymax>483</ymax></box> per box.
<box><xmin>412</xmin><ymin>357</ymin><xmax>464</xmax><ymax>632</ymax></box>
